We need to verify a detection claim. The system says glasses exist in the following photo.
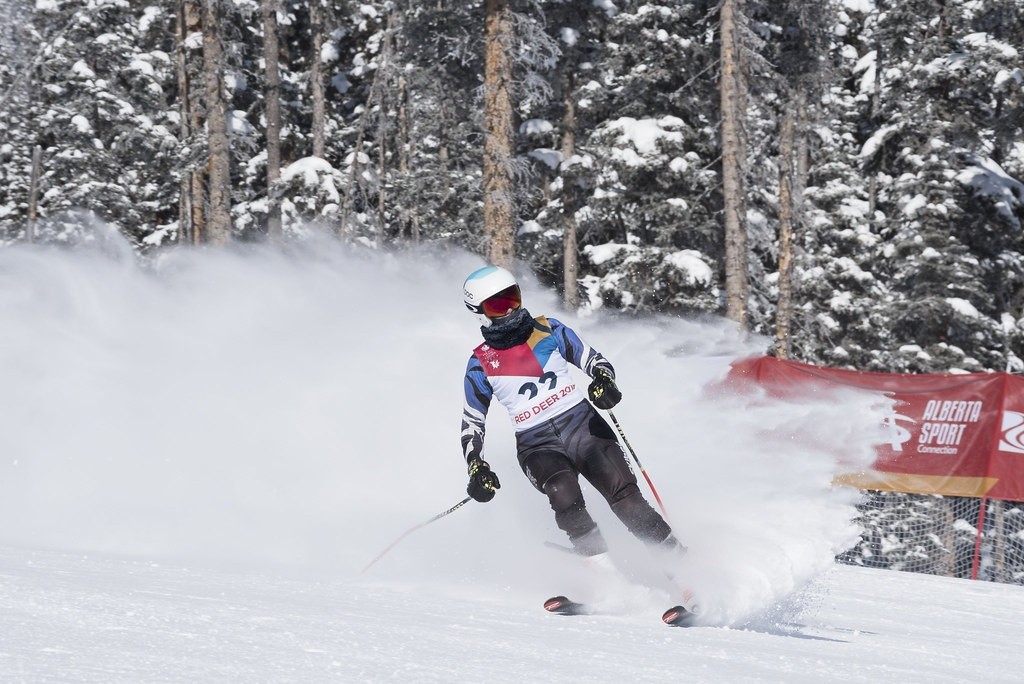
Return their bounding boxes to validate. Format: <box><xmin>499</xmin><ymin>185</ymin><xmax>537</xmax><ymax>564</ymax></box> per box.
<box><xmin>481</xmin><ymin>284</ymin><xmax>521</xmax><ymax>318</ymax></box>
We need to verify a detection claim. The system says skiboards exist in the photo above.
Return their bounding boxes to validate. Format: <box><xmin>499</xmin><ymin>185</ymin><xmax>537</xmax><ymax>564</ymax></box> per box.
<box><xmin>543</xmin><ymin>595</ymin><xmax>708</xmax><ymax>630</ymax></box>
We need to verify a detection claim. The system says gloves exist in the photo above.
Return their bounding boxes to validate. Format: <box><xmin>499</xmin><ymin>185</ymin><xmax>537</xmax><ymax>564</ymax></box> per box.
<box><xmin>588</xmin><ymin>376</ymin><xmax>622</xmax><ymax>410</ymax></box>
<box><xmin>466</xmin><ymin>459</ymin><xmax>500</xmax><ymax>502</ymax></box>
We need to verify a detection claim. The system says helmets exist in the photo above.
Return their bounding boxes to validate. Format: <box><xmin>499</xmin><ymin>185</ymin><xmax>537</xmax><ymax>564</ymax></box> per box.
<box><xmin>463</xmin><ymin>267</ymin><xmax>521</xmax><ymax>328</ymax></box>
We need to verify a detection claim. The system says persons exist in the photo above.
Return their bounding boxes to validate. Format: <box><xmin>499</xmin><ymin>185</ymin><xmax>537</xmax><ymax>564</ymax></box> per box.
<box><xmin>461</xmin><ymin>267</ymin><xmax>701</xmax><ymax>616</ymax></box>
